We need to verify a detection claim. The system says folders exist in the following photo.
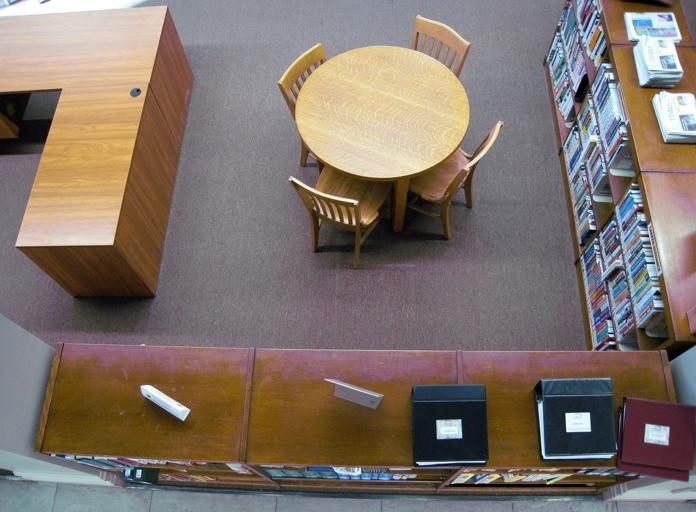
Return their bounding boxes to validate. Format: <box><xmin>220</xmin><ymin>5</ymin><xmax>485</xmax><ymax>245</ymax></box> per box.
<box><xmin>533</xmin><ymin>377</ymin><xmax>618</xmax><ymax>463</ymax></box>
<box><xmin>621</xmin><ymin>396</ymin><xmax>695</xmax><ymax>471</ymax></box>
<box><xmin>614</xmin><ymin>406</ymin><xmax>691</xmax><ymax>482</ymax></box>
<box><xmin>411</xmin><ymin>383</ymin><xmax>489</xmax><ymax>468</ymax></box>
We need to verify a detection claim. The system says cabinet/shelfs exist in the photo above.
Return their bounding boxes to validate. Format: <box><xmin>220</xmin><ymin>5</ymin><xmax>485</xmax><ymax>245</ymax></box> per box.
<box><xmin>543</xmin><ymin>0</ymin><xmax>696</xmax><ymax>361</ymax></box>
<box><xmin>35</xmin><ymin>341</ymin><xmax>679</xmax><ymax>497</ymax></box>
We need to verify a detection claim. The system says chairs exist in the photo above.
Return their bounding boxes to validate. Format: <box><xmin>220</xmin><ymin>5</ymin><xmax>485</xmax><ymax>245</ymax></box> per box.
<box><xmin>407</xmin><ymin>121</ymin><xmax>504</xmax><ymax>240</ymax></box>
<box><xmin>278</xmin><ymin>43</ymin><xmax>327</xmax><ymax>166</ymax></box>
<box><xmin>288</xmin><ymin>166</ymin><xmax>393</xmax><ymax>269</ymax></box>
<box><xmin>412</xmin><ymin>15</ymin><xmax>471</xmax><ymax>78</ymax></box>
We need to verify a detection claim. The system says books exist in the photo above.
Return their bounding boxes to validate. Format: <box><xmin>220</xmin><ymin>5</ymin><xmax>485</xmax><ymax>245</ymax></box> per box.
<box><xmin>576</xmin><ymin>93</ymin><xmax>610</xmax><ymax>197</ymax></box>
<box><xmin>53</xmin><ymin>454</ymin><xmax>252</xmax><ymax>484</ymax></box>
<box><xmin>260</xmin><ymin>466</ymin><xmax>417</xmax><ymax>482</ymax></box>
<box><xmin>634</xmin><ymin>35</ymin><xmax>684</xmax><ymax>89</ymax></box>
<box><xmin>563</xmin><ymin>125</ymin><xmax>597</xmax><ymax>244</ymax></box>
<box><xmin>624</xmin><ymin>12</ymin><xmax>682</xmax><ymax>44</ymax></box>
<box><xmin>449</xmin><ymin>469</ymin><xmax>649</xmax><ymax>485</ymax></box>
<box><xmin>616</xmin><ymin>396</ymin><xmax>696</xmax><ymax>483</ymax></box>
<box><xmin>590</xmin><ymin>64</ymin><xmax>635</xmax><ymax>170</ymax></box>
<box><xmin>598</xmin><ymin>216</ymin><xmax>636</xmax><ymax>343</ymax></box>
<box><xmin>578</xmin><ymin>239</ymin><xmax>616</xmax><ymax>350</ymax></box>
<box><xmin>546</xmin><ymin>32</ymin><xmax>575</xmax><ymax>122</ymax></box>
<box><xmin>576</xmin><ymin>0</ymin><xmax>609</xmax><ymax>71</ymax></box>
<box><xmin>614</xmin><ymin>188</ymin><xmax>667</xmax><ymax>331</ymax></box>
<box><xmin>651</xmin><ymin>90</ymin><xmax>696</xmax><ymax>143</ymax></box>
<box><xmin>560</xmin><ymin>6</ymin><xmax>587</xmax><ymax>100</ymax></box>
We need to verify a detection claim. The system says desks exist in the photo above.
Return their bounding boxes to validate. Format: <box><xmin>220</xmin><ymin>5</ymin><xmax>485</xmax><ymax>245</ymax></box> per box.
<box><xmin>1</xmin><ymin>6</ymin><xmax>196</xmax><ymax>299</ymax></box>
<box><xmin>294</xmin><ymin>46</ymin><xmax>470</xmax><ymax>233</ymax></box>
